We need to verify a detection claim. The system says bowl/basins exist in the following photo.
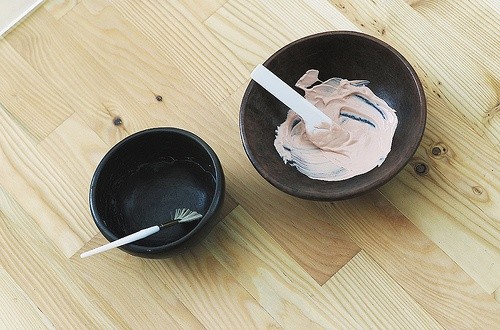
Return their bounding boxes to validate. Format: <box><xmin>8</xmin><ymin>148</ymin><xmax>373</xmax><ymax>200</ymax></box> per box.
<box><xmin>238</xmin><ymin>31</ymin><xmax>427</xmax><ymax>202</ymax></box>
<box><xmin>88</xmin><ymin>127</ymin><xmax>225</xmax><ymax>259</ymax></box>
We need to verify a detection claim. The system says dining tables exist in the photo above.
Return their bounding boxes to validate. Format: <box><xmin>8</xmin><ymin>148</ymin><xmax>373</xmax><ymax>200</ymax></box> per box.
<box><xmin>0</xmin><ymin>1</ymin><xmax>500</xmax><ymax>330</ymax></box>
